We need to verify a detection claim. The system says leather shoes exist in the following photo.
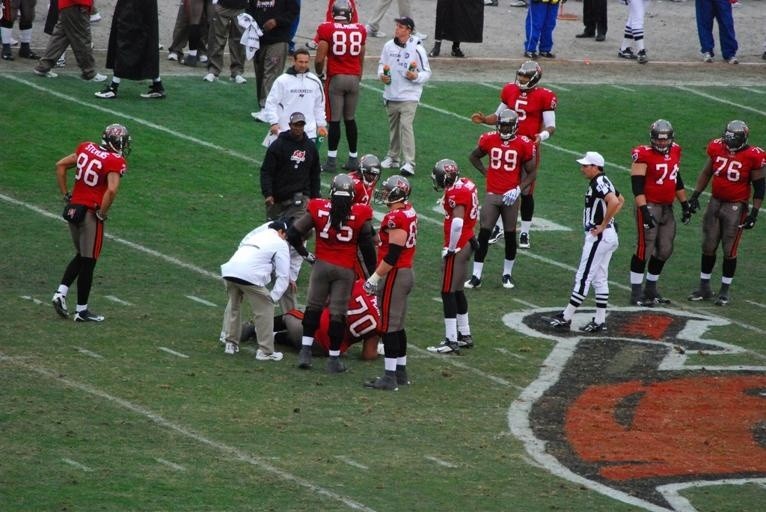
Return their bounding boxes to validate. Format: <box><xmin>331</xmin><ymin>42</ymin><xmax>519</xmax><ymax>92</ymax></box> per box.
<box><xmin>594</xmin><ymin>32</ymin><xmax>606</xmax><ymax>42</ymax></box>
<box><xmin>576</xmin><ymin>31</ymin><xmax>593</xmax><ymax>38</ymax></box>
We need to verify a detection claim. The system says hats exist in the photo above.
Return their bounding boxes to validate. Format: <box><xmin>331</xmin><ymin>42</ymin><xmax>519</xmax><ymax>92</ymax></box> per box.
<box><xmin>289</xmin><ymin>112</ymin><xmax>306</xmax><ymax>125</ymax></box>
<box><xmin>394</xmin><ymin>16</ymin><xmax>416</xmax><ymax>32</ymax></box>
<box><xmin>270</xmin><ymin>219</ymin><xmax>287</xmax><ymax>231</ymax></box>
<box><xmin>575</xmin><ymin>151</ymin><xmax>605</xmax><ymax>167</ymax></box>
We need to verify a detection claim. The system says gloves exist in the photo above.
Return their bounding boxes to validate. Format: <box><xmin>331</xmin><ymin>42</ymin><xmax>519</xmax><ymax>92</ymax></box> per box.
<box><xmin>361</xmin><ymin>273</ymin><xmax>381</xmax><ymax>295</ymax></box>
<box><xmin>303</xmin><ymin>252</ymin><xmax>317</xmax><ymax>264</ymax></box>
<box><xmin>441</xmin><ymin>247</ymin><xmax>461</xmax><ymax>259</ymax></box>
<box><xmin>640</xmin><ymin>203</ymin><xmax>657</xmax><ymax>230</ymax></box>
<box><xmin>678</xmin><ymin>201</ymin><xmax>692</xmax><ymax>226</ymax></box>
<box><xmin>503</xmin><ymin>186</ymin><xmax>522</xmax><ymax>206</ymax></box>
<box><xmin>739</xmin><ymin>210</ymin><xmax>758</xmax><ymax>231</ymax></box>
<box><xmin>690</xmin><ymin>192</ymin><xmax>701</xmax><ymax>214</ymax></box>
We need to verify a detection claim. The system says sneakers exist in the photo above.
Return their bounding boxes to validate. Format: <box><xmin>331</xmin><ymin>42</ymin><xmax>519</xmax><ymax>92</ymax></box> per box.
<box><xmin>450</xmin><ymin>45</ymin><xmax>464</xmax><ymax>57</ymax></box>
<box><xmin>71</xmin><ymin>309</ymin><xmax>107</xmax><ymax>323</ymax></box>
<box><xmin>635</xmin><ymin>50</ymin><xmax>647</xmax><ymax>63</ymax></box>
<box><xmin>363</xmin><ymin>330</ymin><xmax>473</xmax><ymax>391</ymax></box>
<box><xmin>1</xmin><ymin>47</ymin><xmax>12</xmax><ymax>59</ymax></box>
<box><xmin>539</xmin><ymin>310</ymin><xmax>571</xmax><ymax>328</ymax></box>
<box><xmin>462</xmin><ymin>224</ymin><xmax>530</xmax><ymax>290</ymax></box>
<box><xmin>32</xmin><ymin>53</ymin><xmax>166</xmax><ymax>98</ymax></box>
<box><xmin>538</xmin><ymin>50</ymin><xmax>555</xmax><ymax>59</ymax></box>
<box><xmin>725</xmin><ymin>54</ymin><xmax>738</xmax><ymax>64</ymax></box>
<box><xmin>578</xmin><ymin>317</ymin><xmax>607</xmax><ymax>334</ymax></box>
<box><xmin>19</xmin><ymin>48</ymin><xmax>41</xmax><ymax>58</ymax></box>
<box><xmin>321</xmin><ymin>156</ymin><xmax>416</xmax><ymax>174</ymax></box>
<box><xmin>524</xmin><ymin>50</ymin><xmax>539</xmax><ymax>59</ymax></box>
<box><xmin>618</xmin><ymin>46</ymin><xmax>637</xmax><ymax>59</ymax></box>
<box><xmin>52</xmin><ymin>291</ymin><xmax>70</xmax><ymax>319</ymax></box>
<box><xmin>219</xmin><ymin>331</ymin><xmax>345</xmax><ymax>372</ymax></box>
<box><xmin>429</xmin><ymin>46</ymin><xmax>441</xmax><ymax>58</ymax></box>
<box><xmin>630</xmin><ymin>288</ymin><xmax>728</xmax><ymax>307</ymax></box>
<box><xmin>168</xmin><ymin>47</ymin><xmax>248</xmax><ymax>85</ymax></box>
<box><xmin>703</xmin><ymin>50</ymin><xmax>714</xmax><ymax>63</ymax></box>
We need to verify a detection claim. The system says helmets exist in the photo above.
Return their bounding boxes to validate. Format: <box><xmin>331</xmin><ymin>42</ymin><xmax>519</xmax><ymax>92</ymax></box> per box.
<box><xmin>97</xmin><ymin>122</ymin><xmax>132</xmax><ymax>157</ymax></box>
<box><xmin>372</xmin><ymin>174</ymin><xmax>410</xmax><ymax>204</ymax></box>
<box><xmin>650</xmin><ymin>119</ymin><xmax>676</xmax><ymax>155</ymax></box>
<box><xmin>514</xmin><ymin>59</ymin><xmax>543</xmax><ymax>91</ymax></box>
<box><xmin>331</xmin><ymin>0</ymin><xmax>352</xmax><ymax>21</ymax></box>
<box><xmin>496</xmin><ymin>107</ymin><xmax>519</xmax><ymax>141</ymax></box>
<box><xmin>429</xmin><ymin>157</ymin><xmax>457</xmax><ymax>189</ymax></box>
<box><xmin>356</xmin><ymin>152</ymin><xmax>382</xmax><ymax>185</ymax></box>
<box><xmin>331</xmin><ymin>172</ymin><xmax>354</xmax><ymax>197</ymax></box>
<box><xmin>723</xmin><ymin>119</ymin><xmax>750</xmax><ymax>151</ymax></box>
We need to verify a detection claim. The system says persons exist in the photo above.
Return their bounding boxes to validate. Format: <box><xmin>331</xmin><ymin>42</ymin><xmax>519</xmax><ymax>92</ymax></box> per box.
<box><xmin>2</xmin><ymin>1</ymin><xmax>766</xmax><ymax>393</ymax></box>
